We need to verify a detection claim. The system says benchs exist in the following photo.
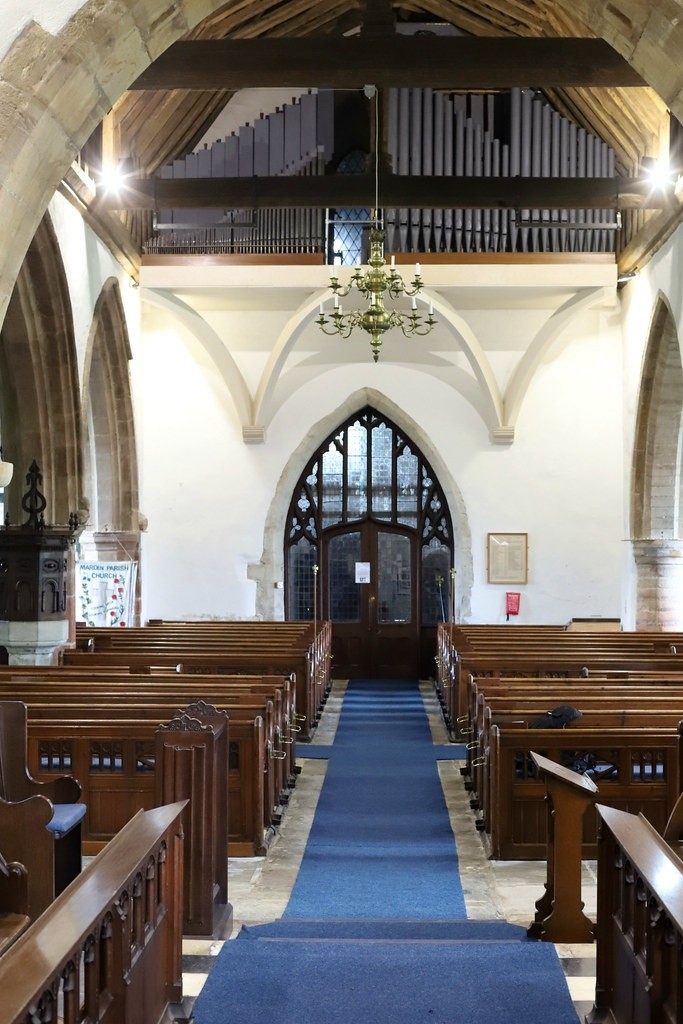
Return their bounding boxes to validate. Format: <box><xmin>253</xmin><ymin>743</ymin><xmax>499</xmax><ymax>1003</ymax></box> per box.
<box><xmin>0</xmin><ymin>620</ymin><xmax>332</xmax><ymax>956</ymax></box>
<box><xmin>434</xmin><ymin>623</ymin><xmax>683</xmax><ymax>860</ymax></box>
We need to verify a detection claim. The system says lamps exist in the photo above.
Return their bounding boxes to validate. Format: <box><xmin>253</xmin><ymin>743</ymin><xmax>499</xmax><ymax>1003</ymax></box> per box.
<box><xmin>315</xmin><ymin>87</ymin><xmax>438</xmax><ymax>363</ymax></box>
